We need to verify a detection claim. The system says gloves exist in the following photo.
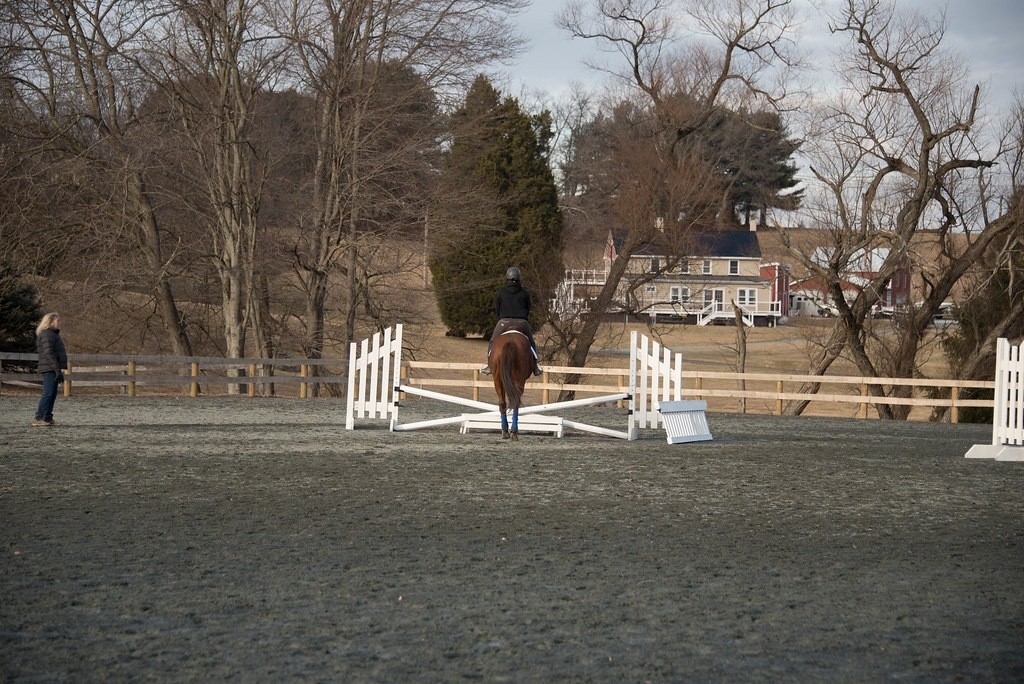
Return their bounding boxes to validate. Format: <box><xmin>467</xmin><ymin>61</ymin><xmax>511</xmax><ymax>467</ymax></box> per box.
<box><xmin>54</xmin><ymin>373</ymin><xmax>65</xmax><ymax>384</ymax></box>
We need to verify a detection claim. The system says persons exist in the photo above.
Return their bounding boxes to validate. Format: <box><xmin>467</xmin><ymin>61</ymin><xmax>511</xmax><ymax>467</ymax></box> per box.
<box><xmin>480</xmin><ymin>266</ymin><xmax>544</xmax><ymax>376</ymax></box>
<box><xmin>31</xmin><ymin>311</ymin><xmax>69</xmax><ymax>426</ymax></box>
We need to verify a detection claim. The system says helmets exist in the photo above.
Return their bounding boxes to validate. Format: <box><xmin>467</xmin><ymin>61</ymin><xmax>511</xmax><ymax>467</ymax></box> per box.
<box><xmin>506</xmin><ymin>267</ymin><xmax>521</xmax><ymax>279</ymax></box>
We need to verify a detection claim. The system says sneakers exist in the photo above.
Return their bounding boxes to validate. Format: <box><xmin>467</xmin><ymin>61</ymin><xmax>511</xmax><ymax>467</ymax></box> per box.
<box><xmin>481</xmin><ymin>366</ymin><xmax>492</xmax><ymax>375</ymax></box>
<box><xmin>31</xmin><ymin>416</ymin><xmax>62</xmax><ymax>427</ymax></box>
<box><xmin>533</xmin><ymin>367</ymin><xmax>543</xmax><ymax>376</ymax></box>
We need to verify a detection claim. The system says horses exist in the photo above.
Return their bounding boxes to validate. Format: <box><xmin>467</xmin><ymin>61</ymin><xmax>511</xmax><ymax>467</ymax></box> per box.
<box><xmin>487</xmin><ymin>329</ymin><xmax>532</xmax><ymax>442</ymax></box>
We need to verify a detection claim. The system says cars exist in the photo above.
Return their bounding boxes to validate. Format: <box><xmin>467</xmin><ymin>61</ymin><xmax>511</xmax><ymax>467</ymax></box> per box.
<box><xmin>873</xmin><ymin>297</ymin><xmax>962</xmax><ymax>328</ymax></box>
<box><xmin>816</xmin><ymin>303</ymin><xmax>869</xmax><ymax>318</ymax></box>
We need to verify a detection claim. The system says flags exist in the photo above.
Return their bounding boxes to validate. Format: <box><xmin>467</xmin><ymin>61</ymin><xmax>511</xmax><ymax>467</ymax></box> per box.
<box><xmin>605</xmin><ymin>232</ymin><xmax>616</xmax><ymax>260</ymax></box>
